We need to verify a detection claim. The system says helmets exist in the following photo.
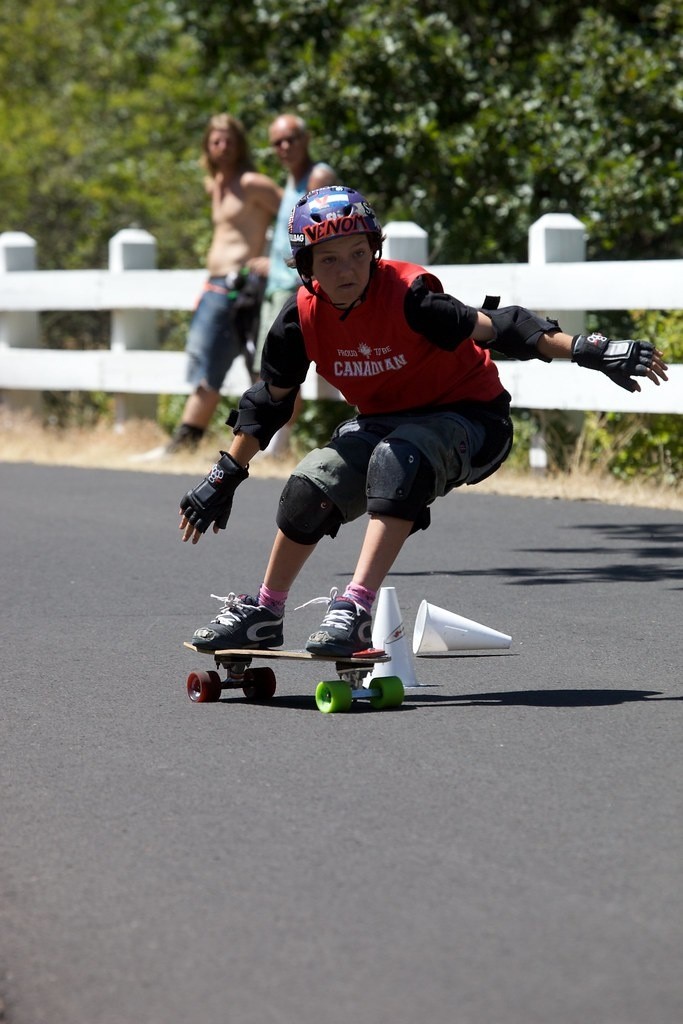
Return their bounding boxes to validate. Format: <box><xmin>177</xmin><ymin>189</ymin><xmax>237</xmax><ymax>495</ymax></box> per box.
<box><xmin>288</xmin><ymin>186</ymin><xmax>381</xmax><ymax>278</ymax></box>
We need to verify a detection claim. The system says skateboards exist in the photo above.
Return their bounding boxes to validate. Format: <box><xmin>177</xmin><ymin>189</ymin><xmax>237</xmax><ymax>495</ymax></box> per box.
<box><xmin>182</xmin><ymin>642</ymin><xmax>404</xmax><ymax>714</ymax></box>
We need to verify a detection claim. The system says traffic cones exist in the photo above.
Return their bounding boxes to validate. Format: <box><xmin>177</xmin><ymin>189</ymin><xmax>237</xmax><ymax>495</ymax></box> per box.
<box><xmin>360</xmin><ymin>585</ymin><xmax>421</xmax><ymax>689</ymax></box>
<box><xmin>412</xmin><ymin>599</ymin><xmax>513</xmax><ymax>656</ymax></box>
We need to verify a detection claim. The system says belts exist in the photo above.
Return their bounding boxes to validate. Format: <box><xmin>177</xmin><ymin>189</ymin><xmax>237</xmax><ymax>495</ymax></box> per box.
<box><xmin>206</xmin><ymin>284</ymin><xmax>230</xmax><ymax>296</ymax></box>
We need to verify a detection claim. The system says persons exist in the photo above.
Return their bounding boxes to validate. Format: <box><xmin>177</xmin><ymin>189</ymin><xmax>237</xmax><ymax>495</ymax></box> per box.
<box><xmin>179</xmin><ymin>184</ymin><xmax>668</xmax><ymax>658</ymax></box>
<box><xmin>253</xmin><ymin>114</ymin><xmax>337</xmax><ymax>458</ymax></box>
<box><xmin>141</xmin><ymin>112</ymin><xmax>284</xmax><ymax>462</ymax></box>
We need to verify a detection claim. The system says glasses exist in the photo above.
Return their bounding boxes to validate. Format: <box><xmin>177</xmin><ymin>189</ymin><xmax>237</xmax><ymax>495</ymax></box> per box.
<box><xmin>274</xmin><ymin>135</ymin><xmax>298</xmax><ymax>146</ymax></box>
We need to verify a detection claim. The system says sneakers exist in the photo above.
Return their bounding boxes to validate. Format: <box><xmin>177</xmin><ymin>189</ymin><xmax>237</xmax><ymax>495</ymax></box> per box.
<box><xmin>192</xmin><ymin>592</ymin><xmax>284</xmax><ymax>648</ymax></box>
<box><xmin>294</xmin><ymin>587</ymin><xmax>373</xmax><ymax>656</ymax></box>
